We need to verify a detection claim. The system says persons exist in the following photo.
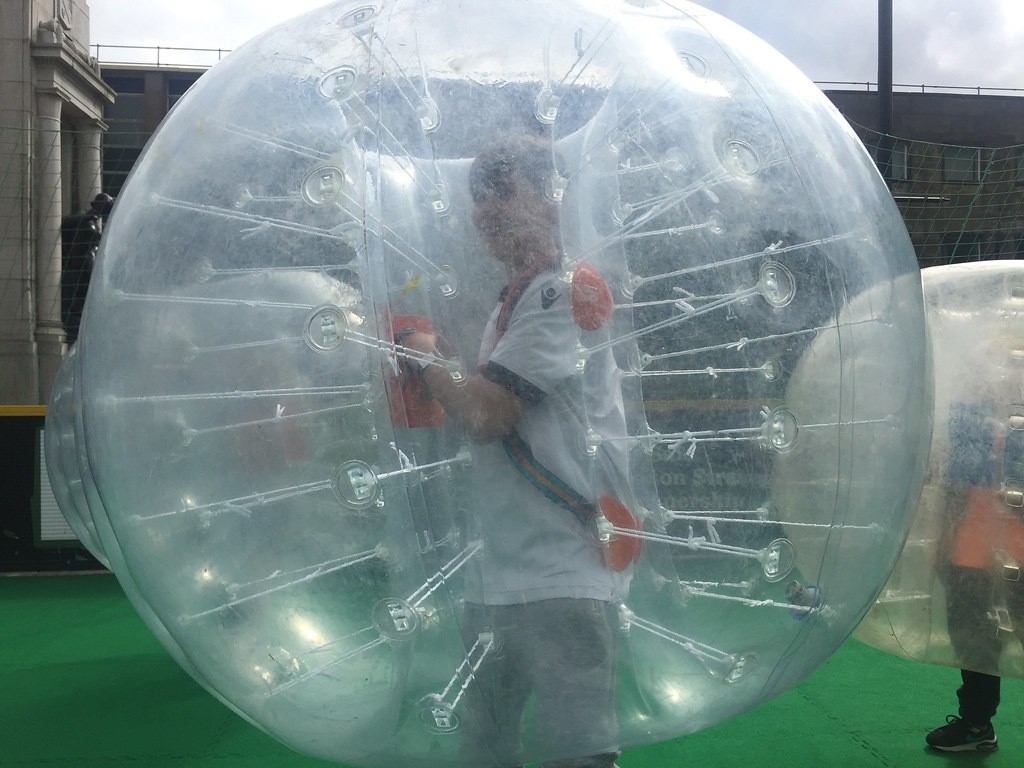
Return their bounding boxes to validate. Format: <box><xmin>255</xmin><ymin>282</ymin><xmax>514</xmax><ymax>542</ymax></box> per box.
<box><xmin>924</xmin><ymin>368</ymin><xmax>1023</xmax><ymax>756</ymax></box>
<box><xmin>59</xmin><ymin>192</ymin><xmax>117</xmax><ymax>358</ymax></box>
<box><xmin>392</xmin><ymin>133</ymin><xmax>641</xmax><ymax>766</ymax></box>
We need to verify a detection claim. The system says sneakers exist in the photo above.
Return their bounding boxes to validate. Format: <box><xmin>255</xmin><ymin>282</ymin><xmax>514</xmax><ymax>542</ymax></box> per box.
<box><xmin>925</xmin><ymin>715</ymin><xmax>1000</xmax><ymax>752</ymax></box>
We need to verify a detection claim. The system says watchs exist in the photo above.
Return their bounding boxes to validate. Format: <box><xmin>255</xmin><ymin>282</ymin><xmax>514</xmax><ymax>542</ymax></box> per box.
<box><xmin>416</xmin><ymin>351</ymin><xmax>443</xmax><ymax>391</ymax></box>
